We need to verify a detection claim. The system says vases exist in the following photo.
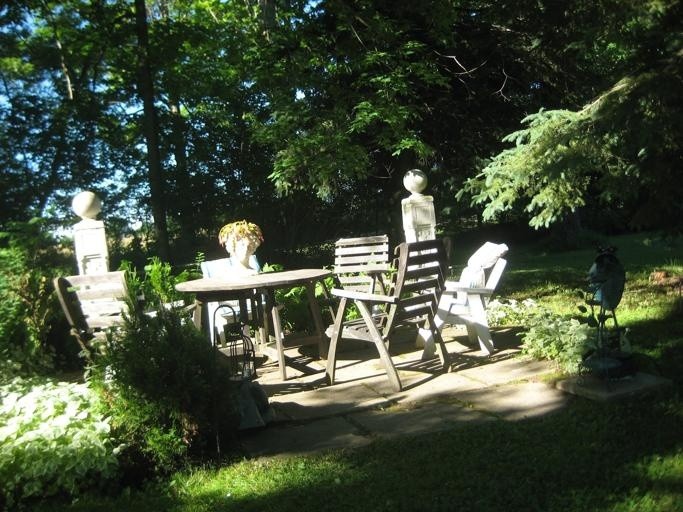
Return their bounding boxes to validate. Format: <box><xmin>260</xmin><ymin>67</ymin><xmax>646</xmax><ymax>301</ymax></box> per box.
<box><xmin>225</xmin><ymin>240</ymin><xmax>261</xmax><ymax>278</ymax></box>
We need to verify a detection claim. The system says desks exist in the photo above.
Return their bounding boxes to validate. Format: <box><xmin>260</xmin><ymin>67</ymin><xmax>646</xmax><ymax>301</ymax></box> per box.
<box><xmin>174</xmin><ymin>266</ymin><xmax>331</xmax><ymax>381</ymax></box>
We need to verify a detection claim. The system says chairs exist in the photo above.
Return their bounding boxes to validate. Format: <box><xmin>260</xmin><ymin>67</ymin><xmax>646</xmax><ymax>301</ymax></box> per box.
<box><xmin>325</xmin><ymin>238</ymin><xmax>452</xmax><ymax>393</ymax></box>
<box><xmin>319</xmin><ymin>232</ymin><xmax>389</xmax><ymax>316</ymax></box>
<box><xmin>198</xmin><ymin>253</ymin><xmax>267</xmax><ymax>349</ymax></box>
<box><xmin>52</xmin><ymin>270</ymin><xmax>134</xmax><ymax>356</ymax></box>
<box><xmin>423</xmin><ymin>258</ymin><xmax>507</xmax><ymax>363</ymax></box>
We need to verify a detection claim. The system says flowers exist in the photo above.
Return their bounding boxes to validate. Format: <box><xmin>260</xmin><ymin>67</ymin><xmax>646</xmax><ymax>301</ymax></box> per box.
<box><xmin>216</xmin><ymin>217</ymin><xmax>269</xmax><ymax>249</ymax></box>
